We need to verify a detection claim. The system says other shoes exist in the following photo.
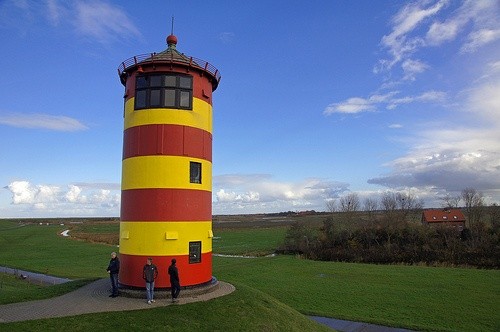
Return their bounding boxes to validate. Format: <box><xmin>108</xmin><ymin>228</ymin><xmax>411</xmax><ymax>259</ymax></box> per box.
<box><xmin>151</xmin><ymin>299</ymin><xmax>156</xmax><ymax>303</ymax></box>
<box><xmin>171</xmin><ymin>298</ymin><xmax>176</xmax><ymax>303</ymax></box>
<box><xmin>109</xmin><ymin>294</ymin><xmax>113</xmax><ymax>297</ymax></box>
<box><xmin>148</xmin><ymin>300</ymin><xmax>152</xmax><ymax>305</ymax></box>
<box><xmin>112</xmin><ymin>294</ymin><xmax>119</xmax><ymax>298</ymax></box>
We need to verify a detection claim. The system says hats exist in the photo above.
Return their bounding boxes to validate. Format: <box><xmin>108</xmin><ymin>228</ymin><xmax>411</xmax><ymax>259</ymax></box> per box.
<box><xmin>111</xmin><ymin>251</ymin><xmax>116</xmax><ymax>255</ymax></box>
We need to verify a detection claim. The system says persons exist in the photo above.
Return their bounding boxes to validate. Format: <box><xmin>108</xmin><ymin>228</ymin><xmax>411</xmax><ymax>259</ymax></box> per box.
<box><xmin>143</xmin><ymin>257</ymin><xmax>158</xmax><ymax>305</ymax></box>
<box><xmin>106</xmin><ymin>251</ymin><xmax>120</xmax><ymax>298</ymax></box>
<box><xmin>168</xmin><ymin>258</ymin><xmax>181</xmax><ymax>303</ymax></box>
